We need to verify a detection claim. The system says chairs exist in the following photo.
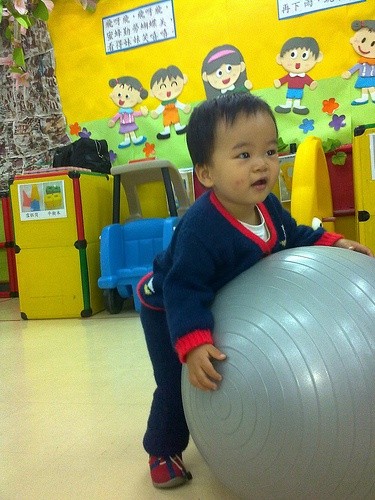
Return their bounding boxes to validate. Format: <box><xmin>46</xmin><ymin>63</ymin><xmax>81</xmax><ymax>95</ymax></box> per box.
<box><xmin>99</xmin><ymin>157</ymin><xmax>181</xmax><ymax>315</ymax></box>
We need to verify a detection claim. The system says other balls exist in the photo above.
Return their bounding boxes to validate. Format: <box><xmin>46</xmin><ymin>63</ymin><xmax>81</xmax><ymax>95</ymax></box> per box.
<box><xmin>164</xmin><ymin>238</ymin><xmax>375</xmax><ymax>500</ymax></box>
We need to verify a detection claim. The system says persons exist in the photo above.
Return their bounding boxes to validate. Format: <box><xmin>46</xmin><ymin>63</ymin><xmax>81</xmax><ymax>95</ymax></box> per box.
<box><xmin>135</xmin><ymin>89</ymin><xmax>373</xmax><ymax>492</ymax></box>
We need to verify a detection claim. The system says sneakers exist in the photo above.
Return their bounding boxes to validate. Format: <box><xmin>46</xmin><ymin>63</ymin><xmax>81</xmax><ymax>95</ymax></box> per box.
<box><xmin>149</xmin><ymin>452</ymin><xmax>186</xmax><ymax>488</ymax></box>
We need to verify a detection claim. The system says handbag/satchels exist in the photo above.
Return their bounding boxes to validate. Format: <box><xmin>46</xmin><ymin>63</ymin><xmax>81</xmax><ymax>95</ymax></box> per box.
<box><xmin>52</xmin><ymin>137</ymin><xmax>112</xmax><ymax>175</ymax></box>
